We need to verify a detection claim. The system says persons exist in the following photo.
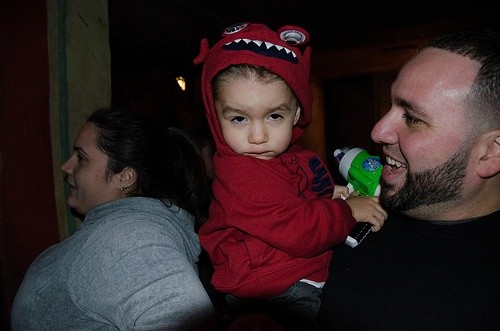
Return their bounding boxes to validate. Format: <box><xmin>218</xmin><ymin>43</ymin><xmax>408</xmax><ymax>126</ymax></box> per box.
<box><xmin>199</xmin><ymin>21</ymin><xmax>388</xmax><ymax>299</ymax></box>
<box><xmin>177</xmin><ymin>34</ymin><xmax>500</xmax><ymax>331</ymax></box>
<box><xmin>12</xmin><ymin>106</ymin><xmax>214</xmax><ymax>331</ymax></box>
